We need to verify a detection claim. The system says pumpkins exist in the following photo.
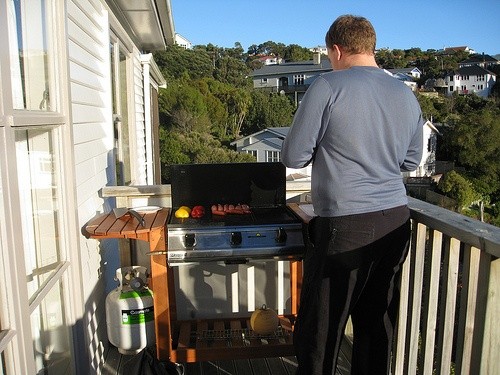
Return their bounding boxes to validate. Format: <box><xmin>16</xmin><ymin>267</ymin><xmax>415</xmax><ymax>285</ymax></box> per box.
<box><xmin>250</xmin><ymin>303</ymin><xmax>278</xmax><ymax>335</ymax></box>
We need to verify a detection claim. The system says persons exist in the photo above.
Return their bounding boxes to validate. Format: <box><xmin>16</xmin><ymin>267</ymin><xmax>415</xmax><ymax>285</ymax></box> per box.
<box><xmin>280</xmin><ymin>14</ymin><xmax>423</xmax><ymax>375</ymax></box>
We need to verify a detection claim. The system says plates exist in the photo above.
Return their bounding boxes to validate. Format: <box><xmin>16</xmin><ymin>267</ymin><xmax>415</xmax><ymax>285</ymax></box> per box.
<box><xmin>130</xmin><ymin>206</ymin><xmax>162</xmax><ymax>215</ymax></box>
